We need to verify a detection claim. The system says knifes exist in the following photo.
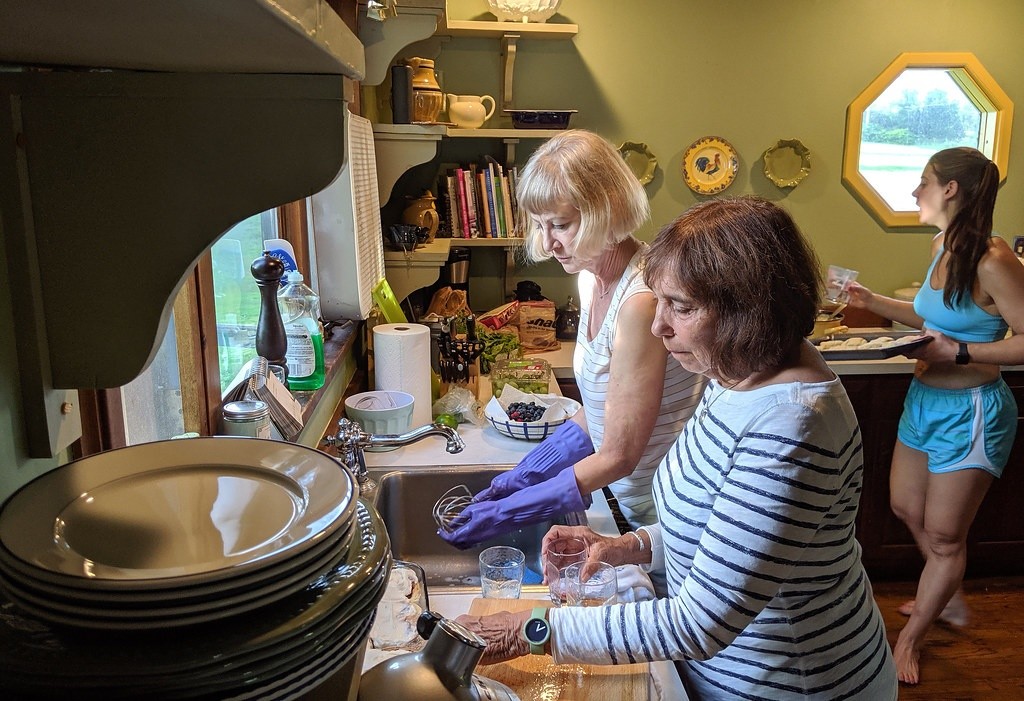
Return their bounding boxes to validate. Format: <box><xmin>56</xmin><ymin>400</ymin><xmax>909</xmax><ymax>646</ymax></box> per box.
<box><xmin>436</xmin><ymin>314</ymin><xmax>485</xmax><ymax>385</ymax></box>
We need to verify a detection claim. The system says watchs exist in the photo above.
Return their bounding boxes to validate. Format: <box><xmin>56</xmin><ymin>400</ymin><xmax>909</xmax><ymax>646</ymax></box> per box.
<box><xmin>956</xmin><ymin>343</ymin><xmax>969</xmax><ymax>365</ymax></box>
<box><xmin>522</xmin><ymin>608</ymin><xmax>551</xmax><ymax>656</ymax></box>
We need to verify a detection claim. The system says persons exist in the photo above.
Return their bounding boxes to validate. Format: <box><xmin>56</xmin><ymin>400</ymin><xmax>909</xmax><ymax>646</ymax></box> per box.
<box><xmin>833</xmin><ymin>147</ymin><xmax>1024</xmax><ymax>686</ymax></box>
<box><xmin>454</xmin><ymin>195</ymin><xmax>897</xmax><ymax>700</ymax></box>
<box><xmin>438</xmin><ymin>128</ymin><xmax>710</xmax><ymax>551</ymax></box>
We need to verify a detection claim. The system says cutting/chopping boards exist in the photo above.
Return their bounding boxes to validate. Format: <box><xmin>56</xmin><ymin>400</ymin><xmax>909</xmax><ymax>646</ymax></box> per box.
<box><xmin>467</xmin><ymin>597</ymin><xmax>652</xmax><ymax>701</ymax></box>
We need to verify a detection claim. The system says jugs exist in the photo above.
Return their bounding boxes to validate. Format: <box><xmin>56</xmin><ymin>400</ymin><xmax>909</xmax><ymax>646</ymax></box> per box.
<box><xmin>400</xmin><ymin>193</ymin><xmax>437</xmax><ymax>242</ymax></box>
<box><xmin>446</xmin><ymin>94</ymin><xmax>496</xmax><ymax>129</ymax></box>
<box><xmin>401</xmin><ymin>57</ymin><xmax>443</xmax><ymax>123</ymax></box>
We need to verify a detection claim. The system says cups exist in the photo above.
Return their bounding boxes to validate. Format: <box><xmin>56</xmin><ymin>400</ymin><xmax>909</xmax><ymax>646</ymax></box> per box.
<box><xmin>354</xmin><ymin>396</ymin><xmax>386</xmax><ymax>410</ymax></box>
<box><xmin>269</xmin><ymin>364</ymin><xmax>286</xmax><ymax>386</ymax></box>
<box><xmin>391</xmin><ymin>65</ymin><xmax>414</xmax><ymax>124</ymax></box>
<box><xmin>546</xmin><ymin>538</ymin><xmax>589</xmax><ymax>608</ymax></box>
<box><xmin>478</xmin><ymin>546</ymin><xmax>525</xmax><ymax>600</ymax></box>
<box><xmin>564</xmin><ymin>561</ymin><xmax>618</xmax><ymax>606</ymax></box>
<box><xmin>823</xmin><ymin>265</ymin><xmax>859</xmax><ymax>306</ymax></box>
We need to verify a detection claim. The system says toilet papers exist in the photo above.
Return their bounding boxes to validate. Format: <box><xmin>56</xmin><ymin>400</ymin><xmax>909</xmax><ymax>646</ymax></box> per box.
<box><xmin>372</xmin><ymin>322</ymin><xmax>433</xmax><ymax>433</ymax></box>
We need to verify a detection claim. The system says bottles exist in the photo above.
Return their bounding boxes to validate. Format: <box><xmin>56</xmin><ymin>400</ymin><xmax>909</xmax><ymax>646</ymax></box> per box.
<box><xmin>276</xmin><ymin>270</ymin><xmax>326</xmax><ymax>390</ymax></box>
<box><xmin>222</xmin><ymin>400</ymin><xmax>271</xmax><ymax>439</ymax></box>
<box><xmin>417</xmin><ymin>312</ymin><xmax>449</xmax><ymax>375</ymax></box>
<box><xmin>556</xmin><ymin>296</ymin><xmax>580</xmax><ymax>339</ymax></box>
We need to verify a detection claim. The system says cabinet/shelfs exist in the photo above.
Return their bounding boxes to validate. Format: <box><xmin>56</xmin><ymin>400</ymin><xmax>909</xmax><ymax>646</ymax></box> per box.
<box><xmin>358</xmin><ymin>0</ymin><xmax>578</xmax><ymax>266</ymax></box>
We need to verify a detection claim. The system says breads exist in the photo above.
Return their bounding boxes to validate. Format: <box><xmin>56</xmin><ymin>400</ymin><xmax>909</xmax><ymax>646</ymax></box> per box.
<box><xmin>814</xmin><ymin>336</ymin><xmax>920</xmax><ymax>350</ymax></box>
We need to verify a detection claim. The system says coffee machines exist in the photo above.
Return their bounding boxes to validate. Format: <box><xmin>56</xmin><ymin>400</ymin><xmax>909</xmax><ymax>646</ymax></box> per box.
<box><xmin>436</xmin><ymin>246</ymin><xmax>471</xmax><ymax>291</ymax></box>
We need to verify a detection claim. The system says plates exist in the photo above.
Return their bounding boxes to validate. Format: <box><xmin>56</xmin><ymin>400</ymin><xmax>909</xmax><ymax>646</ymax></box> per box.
<box><xmin>0</xmin><ymin>436</ymin><xmax>393</xmax><ymax>701</ymax></box>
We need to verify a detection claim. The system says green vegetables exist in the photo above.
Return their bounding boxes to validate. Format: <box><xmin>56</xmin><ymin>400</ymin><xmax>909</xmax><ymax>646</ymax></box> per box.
<box><xmin>454</xmin><ymin>316</ymin><xmax>524</xmax><ymax>374</ymax></box>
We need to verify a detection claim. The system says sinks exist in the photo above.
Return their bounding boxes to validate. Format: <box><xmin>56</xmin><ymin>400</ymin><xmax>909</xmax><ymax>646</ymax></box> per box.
<box><xmin>374</xmin><ymin>470</ymin><xmax>570</xmax><ymax>588</ymax></box>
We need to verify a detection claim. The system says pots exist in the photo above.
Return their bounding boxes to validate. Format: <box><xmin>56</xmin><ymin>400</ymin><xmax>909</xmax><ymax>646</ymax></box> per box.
<box><xmin>806</xmin><ymin>310</ymin><xmax>849</xmax><ymax>339</ymax></box>
<box><xmin>892</xmin><ymin>282</ymin><xmax>922</xmax><ymax>325</ymax></box>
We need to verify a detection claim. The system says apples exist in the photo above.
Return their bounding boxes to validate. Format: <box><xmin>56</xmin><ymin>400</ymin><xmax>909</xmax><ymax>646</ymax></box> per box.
<box><xmin>435</xmin><ymin>415</ymin><xmax>459</xmax><ymax>430</ymax></box>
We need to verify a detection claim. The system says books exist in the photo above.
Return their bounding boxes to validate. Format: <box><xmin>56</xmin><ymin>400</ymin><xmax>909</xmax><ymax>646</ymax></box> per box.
<box><xmin>445</xmin><ymin>155</ymin><xmax>530</xmax><ymax>239</ymax></box>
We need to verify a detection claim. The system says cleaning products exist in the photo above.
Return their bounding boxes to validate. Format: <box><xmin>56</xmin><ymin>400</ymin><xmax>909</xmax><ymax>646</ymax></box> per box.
<box><xmin>276</xmin><ymin>270</ymin><xmax>326</xmax><ymax>391</ymax></box>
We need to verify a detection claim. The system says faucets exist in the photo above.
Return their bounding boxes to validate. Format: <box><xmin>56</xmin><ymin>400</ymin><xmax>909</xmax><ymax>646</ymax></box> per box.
<box><xmin>320</xmin><ymin>418</ymin><xmax>466</xmax><ymax>479</ymax></box>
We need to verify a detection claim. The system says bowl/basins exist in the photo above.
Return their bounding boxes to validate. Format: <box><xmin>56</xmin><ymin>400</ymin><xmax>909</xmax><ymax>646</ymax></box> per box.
<box><xmin>503</xmin><ymin>109</ymin><xmax>579</xmax><ymax>129</ymax></box>
<box><xmin>344</xmin><ymin>390</ymin><xmax>414</xmax><ymax>453</ymax></box>
<box><xmin>484</xmin><ymin>396</ymin><xmax>582</xmax><ymax>440</ymax></box>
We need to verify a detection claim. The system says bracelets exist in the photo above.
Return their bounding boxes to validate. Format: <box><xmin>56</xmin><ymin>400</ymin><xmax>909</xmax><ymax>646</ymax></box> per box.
<box><xmin>627</xmin><ymin>530</ymin><xmax>644</xmax><ymax>551</ymax></box>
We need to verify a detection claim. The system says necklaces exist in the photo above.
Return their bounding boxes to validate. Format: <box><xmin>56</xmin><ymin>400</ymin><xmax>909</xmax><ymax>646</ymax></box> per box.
<box><xmin>597</xmin><ymin>257</ymin><xmax>626</xmax><ymax>299</ymax></box>
<box><xmin>700</xmin><ymin>376</ymin><xmax>745</xmax><ymax>416</ymax></box>
